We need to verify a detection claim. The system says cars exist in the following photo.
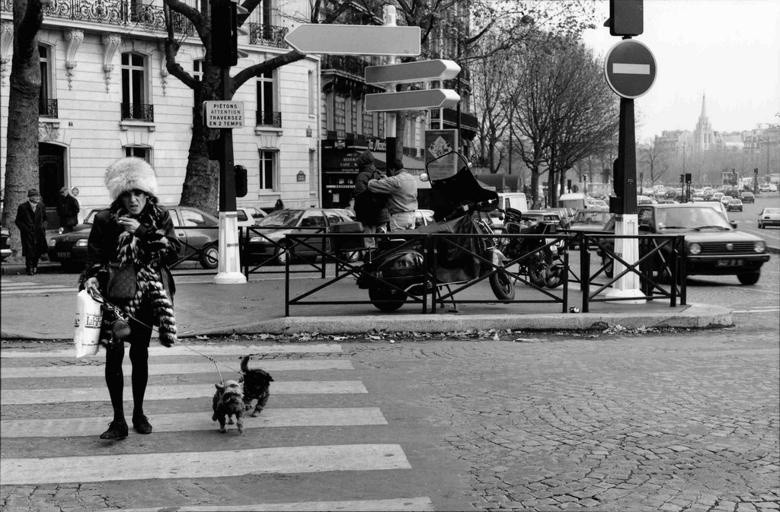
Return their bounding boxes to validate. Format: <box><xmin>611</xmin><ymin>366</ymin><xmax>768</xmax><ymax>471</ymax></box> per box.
<box><xmin>568</xmin><ymin>175</ymin><xmax>780</xmax><ymax>286</ymax></box>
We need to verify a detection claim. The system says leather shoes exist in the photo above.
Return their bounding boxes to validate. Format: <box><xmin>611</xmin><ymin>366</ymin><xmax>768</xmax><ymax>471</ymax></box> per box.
<box><xmin>99</xmin><ymin>418</ymin><xmax>129</xmax><ymax>439</ymax></box>
<box><xmin>132</xmin><ymin>414</ymin><xmax>152</xmax><ymax>434</ymax></box>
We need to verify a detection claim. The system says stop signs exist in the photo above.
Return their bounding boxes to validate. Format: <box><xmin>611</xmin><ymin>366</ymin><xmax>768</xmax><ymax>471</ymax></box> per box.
<box><xmin>602</xmin><ymin>38</ymin><xmax>659</xmax><ymax>101</ymax></box>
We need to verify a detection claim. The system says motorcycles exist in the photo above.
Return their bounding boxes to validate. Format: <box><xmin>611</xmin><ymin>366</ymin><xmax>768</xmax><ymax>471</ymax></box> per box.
<box><xmin>493</xmin><ymin>204</ymin><xmax>567</xmax><ymax>289</ymax></box>
<box><xmin>330</xmin><ymin>146</ymin><xmax>518</xmax><ymax>311</ymax></box>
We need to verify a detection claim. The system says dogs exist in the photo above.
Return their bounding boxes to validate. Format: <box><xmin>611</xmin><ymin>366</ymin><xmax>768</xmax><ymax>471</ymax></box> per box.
<box><xmin>240</xmin><ymin>353</ymin><xmax>274</xmax><ymax>419</ymax></box>
<box><xmin>210</xmin><ymin>376</ymin><xmax>244</xmax><ymax>435</ymax></box>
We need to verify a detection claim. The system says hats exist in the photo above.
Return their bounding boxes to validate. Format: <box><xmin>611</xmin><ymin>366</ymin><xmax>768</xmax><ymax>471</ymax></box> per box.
<box><xmin>27</xmin><ymin>189</ymin><xmax>38</xmax><ymax>197</ymax></box>
<box><xmin>104</xmin><ymin>156</ymin><xmax>160</xmax><ymax>203</ymax></box>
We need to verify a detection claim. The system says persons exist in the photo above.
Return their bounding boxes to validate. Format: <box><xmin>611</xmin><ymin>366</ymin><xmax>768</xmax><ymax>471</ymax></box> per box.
<box><xmin>78</xmin><ymin>157</ymin><xmax>177</xmax><ymax>441</ymax></box>
<box><xmin>57</xmin><ymin>187</ymin><xmax>81</xmax><ymax>231</ymax></box>
<box><xmin>367</xmin><ymin>158</ymin><xmax>422</xmax><ymax>235</ymax></box>
<box><xmin>16</xmin><ymin>188</ymin><xmax>55</xmax><ymax>271</ymax></box>
<box><xmin>353</xmin><ymin>149</ymin><xmax>391</xmax><ymax>262</ymax></box>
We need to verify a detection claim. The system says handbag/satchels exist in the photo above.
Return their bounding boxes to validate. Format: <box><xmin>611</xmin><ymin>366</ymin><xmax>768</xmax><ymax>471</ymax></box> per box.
<box><xmin>74</xmin><ymin>284</ymin><xmax>103</xmax><ymax>360</ymax></box>
